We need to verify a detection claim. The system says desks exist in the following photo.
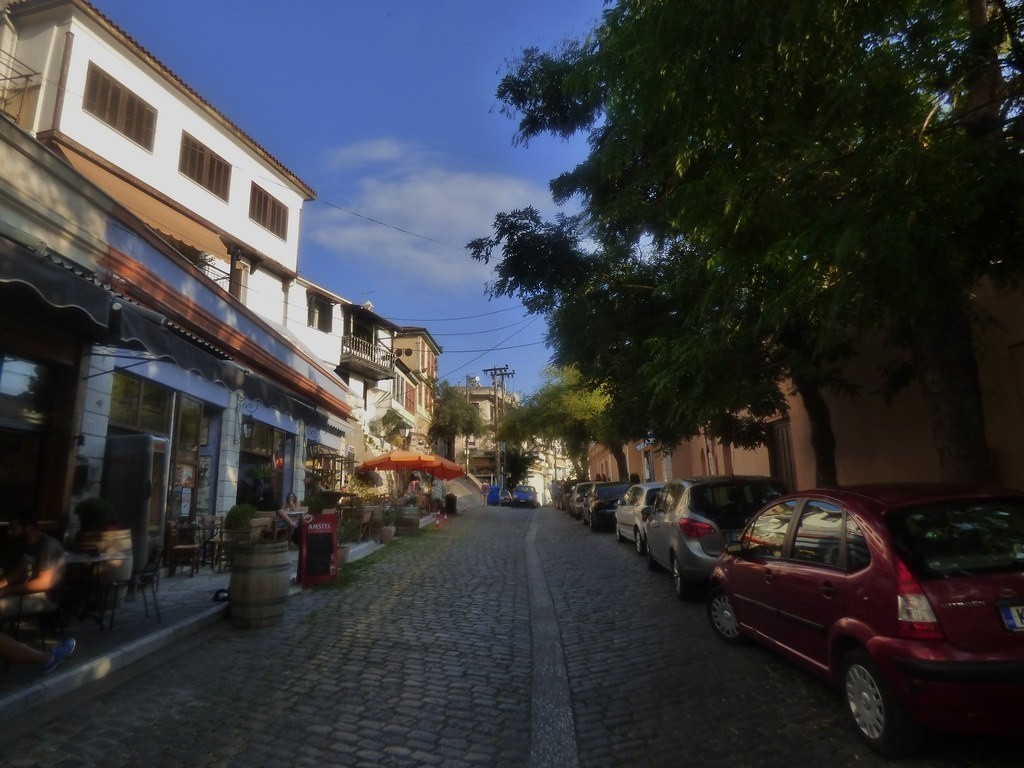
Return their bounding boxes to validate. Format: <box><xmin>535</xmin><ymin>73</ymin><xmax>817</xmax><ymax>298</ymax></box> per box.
<box><xmin>283</xmin><ymin>509</ymin><xmax>305</xmax><ymax>516</ymax></box>
<box><xmin>180</xmin><ymin>523</ymin><xmax>221</xmax><ymax>566</ymax></box>
<box><xmin>69</xmin><ymin>548</ymin><xmax>130</xmax><ymax>630</ymax></box>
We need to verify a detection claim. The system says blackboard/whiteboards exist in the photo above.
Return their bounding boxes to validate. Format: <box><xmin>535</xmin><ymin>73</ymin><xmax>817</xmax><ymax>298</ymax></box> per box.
<box><xmin>297</xmin><ymin>512</ymin><xmax>340</xmax><ymax>587</ymax></box>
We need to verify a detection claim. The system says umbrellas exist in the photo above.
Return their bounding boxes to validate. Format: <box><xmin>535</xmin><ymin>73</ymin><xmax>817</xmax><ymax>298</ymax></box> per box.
<box><xmin>360</xmin><ymin>450</ymin><xmax>434</xmax><ymax>470</ymax></box>
<box><xmin>427</xmin><ymin>454</ymin><xmax>464</xmax><ymax>481</ymax></box>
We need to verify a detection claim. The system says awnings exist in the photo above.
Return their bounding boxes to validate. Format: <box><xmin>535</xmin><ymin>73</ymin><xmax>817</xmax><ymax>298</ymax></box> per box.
<box><xmin>0</xmin><ymin>234</ymin><xmax>329</xmax><ymax>432</ymax></box>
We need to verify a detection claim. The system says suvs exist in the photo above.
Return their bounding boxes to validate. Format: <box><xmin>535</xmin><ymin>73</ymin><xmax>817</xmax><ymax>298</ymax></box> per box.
<box><xmin>614</xmin><ymin>480</ymin><xmax>666</xmax><ymax>556</ymax></box>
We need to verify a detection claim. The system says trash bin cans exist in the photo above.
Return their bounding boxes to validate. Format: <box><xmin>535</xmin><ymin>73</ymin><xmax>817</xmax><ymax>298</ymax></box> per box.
<box><xmin>445</xmin><ymin>494</ymin><xmax>457</xmax><ymax>515</ymax></box>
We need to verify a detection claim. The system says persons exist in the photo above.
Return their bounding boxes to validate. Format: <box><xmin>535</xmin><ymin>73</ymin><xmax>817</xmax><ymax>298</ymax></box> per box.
<box><xmin>407</xmin><ymin>480</ymin><xmax>419</xmax><ymax>493</ymax></box>
<box><xmin>0</xmin><ymin>510</ymin><xmax>68</xmax><ymax>619</ymax></box>
<box><xmin>251</xmin><ymin>488</ymin><xmax>298</xmax><ymax>542</ymax></box>
<box><xmin>0</xmin><ymin>631</ymin><xmax>76</xmax><ymax>673</ymax></box>
<box><xmin>481</xmin><ymin>480</ymin><xmax>489</xmax><ymax>506</ymax></box>
<box><xmin>282</xmin><ymin>493</ymin><xmax>299</xmax><ymax>548</ymax></box>
<box><xmin>548</xmin><ymin>480</ymin><xmax>560</xmax><ymax>510</ymax></box>
<box><xmin>430</xmin><ymin>480</ymin><xmax>449</xmax><ymax>519</ymax></box>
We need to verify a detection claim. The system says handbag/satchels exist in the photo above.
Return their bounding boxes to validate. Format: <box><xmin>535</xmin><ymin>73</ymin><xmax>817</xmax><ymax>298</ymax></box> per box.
<box><xmin>480</xmin><ymin>487</ymin><xmax>484</xmax><ymax>494</ymax></box>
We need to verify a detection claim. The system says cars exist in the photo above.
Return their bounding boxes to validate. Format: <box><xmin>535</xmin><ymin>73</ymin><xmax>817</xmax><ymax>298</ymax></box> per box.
<box><xmin>500</xmin><ymin>490</ymin><xmax>512</xmax><ymax>506</ymax></box>
<box><xmin>580</xmin><ymin>482</ymin><xmax>634</xmax><ymax>532</ymax></box>
<box><xmin>707</xmin><ymin>482</ymin><xmax>1024</xmax><ymax>761</ymax></box>
<box><xmin>562</xmin><ymin>479</ymin><xmax>604</xmax><ymax>521</ymax></box>
<box><xmin>511</xmin><ymin>485</ymin><xmax>537</xmax><ymax>509</ymax></box>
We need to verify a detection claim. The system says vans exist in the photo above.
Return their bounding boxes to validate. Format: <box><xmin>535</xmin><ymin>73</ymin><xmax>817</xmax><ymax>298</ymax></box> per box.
<box><xmin>640</xmin><ymin>477</ymin><xmax>787</xmax><ymax>602</ymax></box>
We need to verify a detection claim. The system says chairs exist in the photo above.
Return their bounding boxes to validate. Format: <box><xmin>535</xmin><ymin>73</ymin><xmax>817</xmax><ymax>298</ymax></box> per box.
<box><xmin>7</xmin><ymin>493</ymin><xmax>387</xmax><ymax>641</ymax></box>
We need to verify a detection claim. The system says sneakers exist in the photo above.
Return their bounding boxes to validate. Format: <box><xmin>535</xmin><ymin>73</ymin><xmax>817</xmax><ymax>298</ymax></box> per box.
<box><xmin>37</xmin><ymin>637</ymin><xmax>76</xmax><ymax>674</ymax></box>
